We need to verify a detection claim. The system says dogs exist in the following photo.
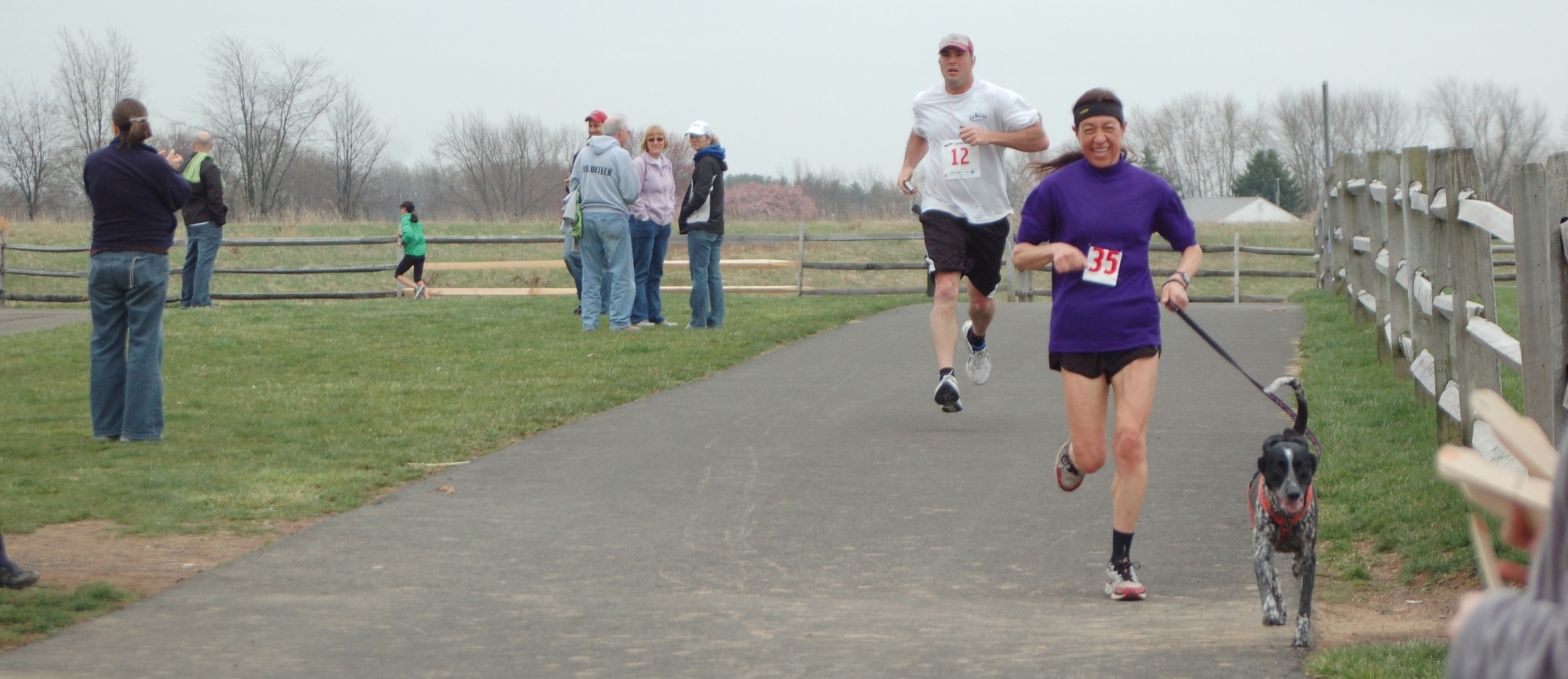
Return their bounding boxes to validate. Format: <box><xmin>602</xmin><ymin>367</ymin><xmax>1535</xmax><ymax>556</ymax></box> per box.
<box><xmin>1249</xmin><ymin>377</ymin><xmax>1319</xmax><ymax>648</ymax></box>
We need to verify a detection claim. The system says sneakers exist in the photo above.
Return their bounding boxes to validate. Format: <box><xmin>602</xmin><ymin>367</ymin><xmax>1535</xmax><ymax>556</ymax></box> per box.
<box><xmin>1105</xmin><ymin>545</ymin><xmax>1147</xmax><ymax>600</ymax></box>
<box><xmin>962</xmin><ymin>319</ymin><xmax>991</xmax><ymax>385</ymax></box>
<box><xmin>934</xmin><ymin>370</ymin><xmax>963</xmax><ymax>413</ymax></box>
<box><xmin>1055</xmin><ymin>438</ymin><xmax>1086</xmax><ymax>492</ymax></box>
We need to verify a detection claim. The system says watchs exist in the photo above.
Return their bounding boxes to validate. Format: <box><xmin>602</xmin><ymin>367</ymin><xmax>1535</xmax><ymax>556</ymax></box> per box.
<box><xmin>1171</xmin><ymin>270</ymin><xmax>1190</xmax><ymax>287</ymax></box>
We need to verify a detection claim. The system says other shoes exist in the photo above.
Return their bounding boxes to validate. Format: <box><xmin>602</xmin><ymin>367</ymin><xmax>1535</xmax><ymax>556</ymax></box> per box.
<box><xmin>414</xmin><ymin>285</ymin><xmax>425</xmax><ymax>300</ymax></box>
<box><xmin>661</xmin><ymin>319</ymin><xmax>678</xmax><ymax>327</ymax></box>
<box><xmin>181</xmin><ymin>305</ymin><xmax>221</xmax><ymax>310</ymax></box>
<box><xmin>621</xmin><ymin>326</ymin><xmax>640</xmax><ymax>330</ymax></box>
<box><xmin>0</xmin><ymin>566</ymin><xmax>39</xmax><ymax>590</ymax></box>
<box><xmin>120</xmin><ymin>434</ymin><xmax>167</xmax><ymax>443</ymax></box>
<box><xmin>574</xmin><ymin>305</ymin><xmax>583</xmax><ymax>314</ymax></box>
<box><xmin>685</xmin><ymin>323</ymin><xmax>708</xmax><ymax>329</ymax></box>
<box><xmin>634</xmin><ymin>320</ymin><xmax>654</xmax><ymax>326</ymax></box>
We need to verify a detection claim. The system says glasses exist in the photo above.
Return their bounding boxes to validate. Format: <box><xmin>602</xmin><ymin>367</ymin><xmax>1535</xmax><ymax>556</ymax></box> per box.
<box><xmin>647</xmin><ymin>137</ymin><xmax>664</xmax><ymax>142</ymax></box>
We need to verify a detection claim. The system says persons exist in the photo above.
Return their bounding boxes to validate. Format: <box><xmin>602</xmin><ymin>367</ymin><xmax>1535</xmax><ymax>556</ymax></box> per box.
<box><xmin>561</xmin><ymin>111</ymin><xmax>610</xmax><ymax>314</ymax></box>
<box><xmin>1014</xmin><ymin>87</ymin><xmax>1203</xmax><ymax>600</ymax></box>
<box><xmin>394</xmin><ymin>201</ymin><xmax>431</xmax><ymax>300</ymax></box>
<box><xmin>679</xmin><ymin>122</ymin><xmax>728</xmax><ymax>329</ymax></box>
<box><xmin>1448</xmin><ymin>418</ymin><xmax>1568</xmax><ymax>679</ymax></box>
<box><xmin>82</xmin><ymin>97</ymin><xmax>192</xmax><ymax>439</ymax></box>
<box><xmin>176</xmin><ymin>130</ymin><xmax>228</xmax><ymax>310</ymax></box>
<box><xmin>0</xmin><ymin>529</ymin><xmax>39</xmax><ymax>588</ymax></box>
<box><xmin>897</xmin><ymin>32</ymin><xmax>1048</xmax><ymax>413</ymax></box>
<box><xmin>562</xmin><ymin>115</ymin><xmax>641</xmax><ymax>331</ymax></box>
<box><xmin>629</xmin><ymin>125</ymin><xmax>678</xmax><ymax>328</ymax></box>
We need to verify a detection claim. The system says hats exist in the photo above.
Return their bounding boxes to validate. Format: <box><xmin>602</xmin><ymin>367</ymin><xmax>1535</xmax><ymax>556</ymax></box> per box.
<box><xmin>684</xmin><ymin>120</ymin><xmax>714</xmax><ymax>137</ymax></box>
<box><xmin>939</xmin><ymin>33</ymin><xmax>974</xmax><ymax>54</ymax></box>
<box><xmin>585</xmin><ymin>111</ymin><xmax>607</xmax><ymax>123</ymax></box>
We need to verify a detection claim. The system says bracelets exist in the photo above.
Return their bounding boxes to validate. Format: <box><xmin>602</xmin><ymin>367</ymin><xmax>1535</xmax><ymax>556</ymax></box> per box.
<box><xmin>1163</xmin><ymin>279</ymin><xmax>1188</xmax><ymax>291</ymax></box>
